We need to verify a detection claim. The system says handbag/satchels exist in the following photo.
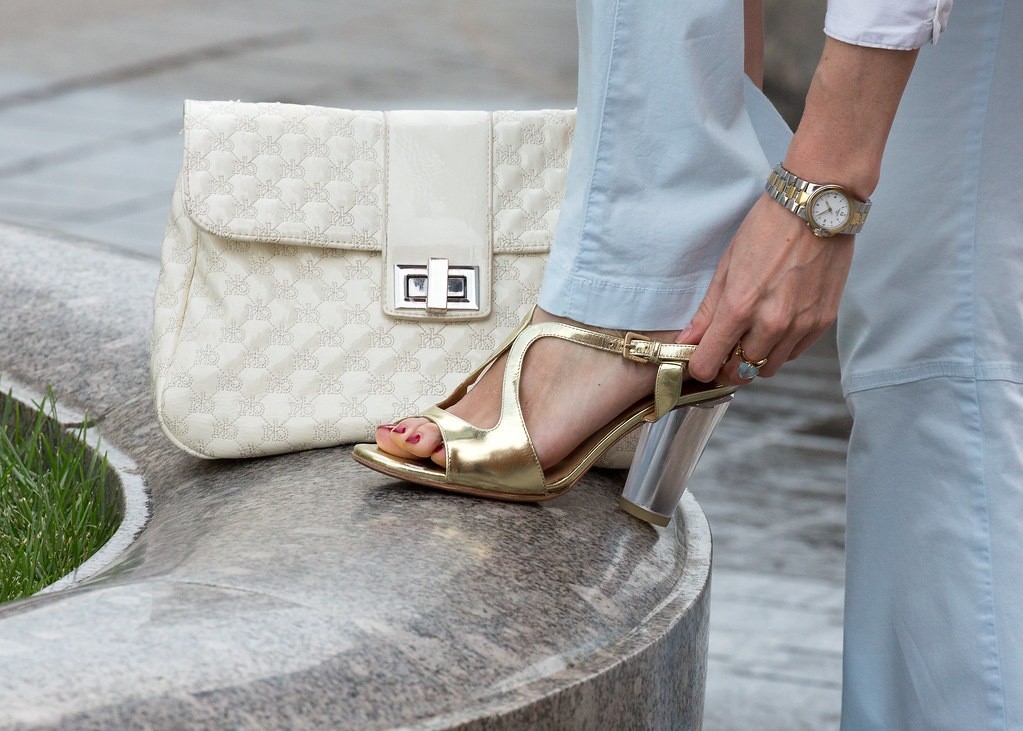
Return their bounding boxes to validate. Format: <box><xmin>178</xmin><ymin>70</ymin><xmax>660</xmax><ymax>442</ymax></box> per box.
<box><xmin>146</xmin><ymin>99</ymin><xmax>579</xmax><ymax>460</ymax></box>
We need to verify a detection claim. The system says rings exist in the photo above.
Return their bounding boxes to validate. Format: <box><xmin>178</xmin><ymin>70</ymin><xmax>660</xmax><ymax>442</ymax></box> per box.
<box><xmin>735</xmin><ymin>340</ymin><xmax>769</xmax><ymax>380</ymax></box>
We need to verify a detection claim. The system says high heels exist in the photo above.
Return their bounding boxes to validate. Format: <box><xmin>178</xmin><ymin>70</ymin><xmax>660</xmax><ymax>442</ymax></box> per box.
<box><xmin>350</xmin><ymin>302</ymin><xmax>739</xmax><ymax>527</ymax></box>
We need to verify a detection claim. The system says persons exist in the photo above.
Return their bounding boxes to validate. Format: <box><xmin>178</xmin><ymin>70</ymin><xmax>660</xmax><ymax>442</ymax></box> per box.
<box><xmin>347</xmin><ymin>0</ymin><xmax>1023</xmax><ymax>731</ymax></box>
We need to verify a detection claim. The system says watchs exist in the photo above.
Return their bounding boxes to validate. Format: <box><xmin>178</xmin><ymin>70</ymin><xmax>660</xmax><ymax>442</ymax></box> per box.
<box><xmin>766</xmin><ymin>163</ymin><xmax>869</xmax><ymax>242</ymax></box>
